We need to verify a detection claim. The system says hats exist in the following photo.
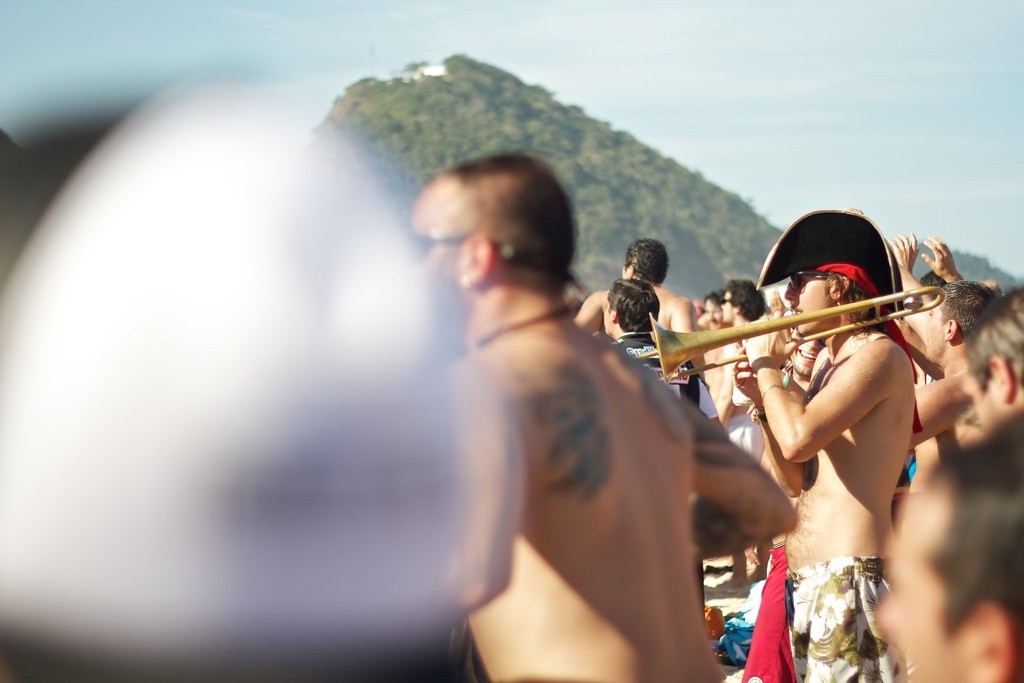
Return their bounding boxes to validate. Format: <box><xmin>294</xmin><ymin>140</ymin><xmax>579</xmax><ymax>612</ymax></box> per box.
<box><xmin>755</xmin><ymin>207</ymin><xmax>909</xmax><ymax>323</ymax></box>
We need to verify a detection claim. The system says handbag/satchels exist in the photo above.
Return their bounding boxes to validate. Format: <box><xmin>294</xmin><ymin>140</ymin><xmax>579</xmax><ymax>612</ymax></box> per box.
<box><xmin>705</xmin><ymin>605</ymin><xmax>726</xmax><ymax>640</ymax></box>
<box><xmin>712</xmin><ymin>617</ymin><xmax>755</xmax><ymax>669</ymax></box>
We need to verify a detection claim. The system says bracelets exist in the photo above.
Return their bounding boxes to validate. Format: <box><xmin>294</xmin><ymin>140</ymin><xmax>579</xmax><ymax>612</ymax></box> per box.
<box><xmin>762</xmin><ymin>384</ymin><xmax>785</xmax><ymax>399</ymax></box>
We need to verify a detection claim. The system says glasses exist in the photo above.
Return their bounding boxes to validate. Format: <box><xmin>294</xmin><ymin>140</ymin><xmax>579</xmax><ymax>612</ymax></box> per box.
<box><xmin>413</xmin><ymin>233</ymin><xmax>464</xmax><ymax>254</ymax></box>
<box><xmin>719</xmin><ymin>298</ymin><xmax>731</xmax><ymax>304</ymax></box>
<box><xmin>787</xmin><ymin>270</ymin><xmax>828</xmax><ymax>292</ymax></box>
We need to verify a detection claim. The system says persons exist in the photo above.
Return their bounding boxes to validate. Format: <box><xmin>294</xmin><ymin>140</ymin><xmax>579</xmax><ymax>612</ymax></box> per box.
<box><xmin>0</xmin><ymin>86</ymin><xmax>1024</xmax><ymax>683</ymax></box>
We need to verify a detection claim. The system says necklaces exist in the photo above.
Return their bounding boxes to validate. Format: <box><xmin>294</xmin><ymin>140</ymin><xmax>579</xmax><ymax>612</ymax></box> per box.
<box><xmin>476</xmin><ymin>304</ymin><xmax>568</xmax><ymax>349</ymax></box>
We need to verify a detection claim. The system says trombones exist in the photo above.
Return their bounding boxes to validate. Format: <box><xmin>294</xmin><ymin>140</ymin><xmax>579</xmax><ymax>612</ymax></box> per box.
<box><xmin>629</xmin><ymin>285</ymin><xmax>947</xmax><ymax>382</ymax></box>
